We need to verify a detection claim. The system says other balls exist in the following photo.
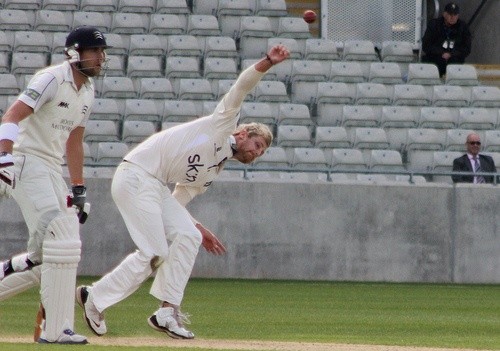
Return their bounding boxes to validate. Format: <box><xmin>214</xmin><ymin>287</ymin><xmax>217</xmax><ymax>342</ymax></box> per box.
<box><xmin>302</xmin><ymin>10</ymin><xmax>318</xmax><ymax>24</ymax></box>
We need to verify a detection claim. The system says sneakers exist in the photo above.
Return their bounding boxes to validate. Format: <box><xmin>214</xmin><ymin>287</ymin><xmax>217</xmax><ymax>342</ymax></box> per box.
<box><xmin>38</xmin><ymin>320</ymin><xmax>88</xmax><ymax>345</ymax></box>
<box><xmin>76</xmin><ymin>285</ymin><xmax>107</xmax><ymax>337</ymax></box>
<box><xmin>147</xmin><ymin>306</ymin><xmax>194</xmax><ymax>339</ymax></box>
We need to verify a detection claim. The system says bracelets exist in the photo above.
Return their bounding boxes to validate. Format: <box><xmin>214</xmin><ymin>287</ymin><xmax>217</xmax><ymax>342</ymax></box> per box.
<box><xmin>71</xmin><ymin>177</ymin><xmax>83</xmax><ymax>185</ymax></box>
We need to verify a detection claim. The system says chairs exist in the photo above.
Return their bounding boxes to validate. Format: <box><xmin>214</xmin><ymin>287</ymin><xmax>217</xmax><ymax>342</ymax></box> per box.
<box><xmin>0</xmin><ymin>0</ymin><xmax>500</xmax><ymax>184</ymax></box>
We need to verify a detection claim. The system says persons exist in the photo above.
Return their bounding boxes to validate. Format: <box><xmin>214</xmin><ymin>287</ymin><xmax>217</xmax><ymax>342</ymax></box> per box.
<box><xmin>75</xmin><ymin>43</ymin><xmax>291</xmax><ymax>339</ymax></box>
<box><xmin>452</xmin><ymin>133</ymin><xmax>500</xmax><ymax>184</ymax></box>
<box><xmin>420</xmin><ymin>3</ymin><xmax>470</xmax><ymax>78</ymax></box>
<box><xmin>0</xmin><ymin>27</ymin><xmax>113</xmax><ymax>344</ymax></box>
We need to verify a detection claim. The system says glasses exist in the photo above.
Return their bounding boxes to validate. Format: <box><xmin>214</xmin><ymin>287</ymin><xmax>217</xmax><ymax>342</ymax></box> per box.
<box><xmin>469</xmin><ymin>141</ymin><xmax>482</xmax><ymax>146</ymax></box>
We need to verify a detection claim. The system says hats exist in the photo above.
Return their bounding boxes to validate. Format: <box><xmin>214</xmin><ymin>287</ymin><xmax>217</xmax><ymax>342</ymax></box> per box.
<box><xmin>445</xmin><ymin>2</ymin><xmax>458</xmax><ymax>16</ymax></box>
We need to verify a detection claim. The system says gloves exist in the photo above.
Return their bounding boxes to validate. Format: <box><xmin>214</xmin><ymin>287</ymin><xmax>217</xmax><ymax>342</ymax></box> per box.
<box><xmin>73</xmin><ymin>185</ymin><xmax>91</xmax><ymax>225</ymax></box>
<box><xmin>0</xmin><ymin>154</ymin><xmax>14</xmax><ymax>199</ymax></box>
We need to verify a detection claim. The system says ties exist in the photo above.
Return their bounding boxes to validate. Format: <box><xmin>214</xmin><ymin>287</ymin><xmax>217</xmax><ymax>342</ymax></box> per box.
<box><xmin>473</xmin><ymin>157</ymin><xmax>486</xmax><ymax>184</ymax></box>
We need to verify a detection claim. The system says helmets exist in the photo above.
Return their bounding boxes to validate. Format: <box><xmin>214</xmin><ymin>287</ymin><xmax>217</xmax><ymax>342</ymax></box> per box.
<box><xmin>65</xmin><ymin>26</ymin><xmax>114</xmax><ymax>56</ymax></box>
